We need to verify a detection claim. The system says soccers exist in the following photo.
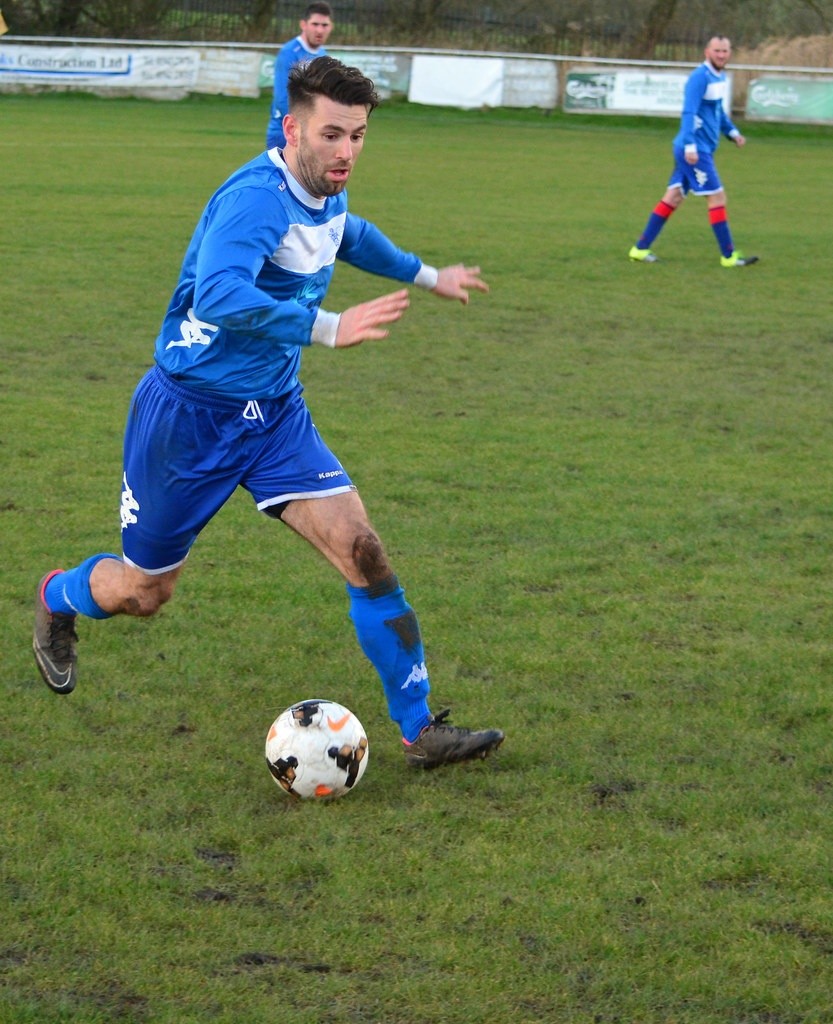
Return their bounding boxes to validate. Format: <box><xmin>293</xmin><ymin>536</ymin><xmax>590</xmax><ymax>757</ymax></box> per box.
<box><xmin>265</xmin><ymin>698</ymin><xmax>369</xmax><ymax>801</ymax></box>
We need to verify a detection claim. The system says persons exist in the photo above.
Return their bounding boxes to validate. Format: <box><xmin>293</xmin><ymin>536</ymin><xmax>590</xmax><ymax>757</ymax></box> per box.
<box><xmin>32</xmin><ymin>58</ymin><xmax>506</xmax><ymax>774</ymax></box>
<box><xmin>628</xmin><ymin>35</ymin><xmax>760</xmax><ymax>267</ymax></box>
<box><xmin>266</xmin><ymin>2</ymin><xmax>334</xmax><ymax>149</ymax></box>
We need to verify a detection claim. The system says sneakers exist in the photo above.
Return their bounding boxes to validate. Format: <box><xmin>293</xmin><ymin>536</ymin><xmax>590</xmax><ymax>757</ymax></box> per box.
<box><xmin>32</xmin><ymin>568</ymin><xmax>76</xmax><ymax>694</ymax></box>
<box><xmin>720</xmin><ymin>250</ymin><xmax>757</xmax><ymax>267</ymax></box>
<box><xmin>629</xmin><ymin>246</ymin><xmax>661</xmax><ymax>262</ymax></box>
<box><xmin>401</xmin><ymin>710</ymin><xmax>503</xmax><ymax>772</ymax></box>
